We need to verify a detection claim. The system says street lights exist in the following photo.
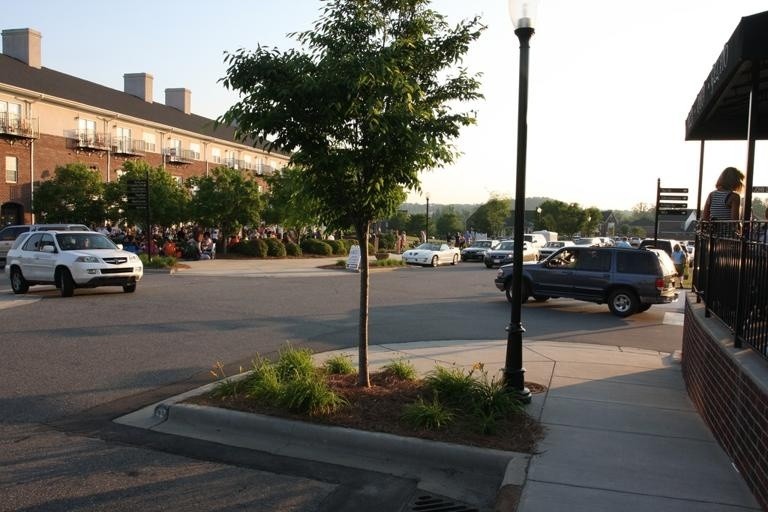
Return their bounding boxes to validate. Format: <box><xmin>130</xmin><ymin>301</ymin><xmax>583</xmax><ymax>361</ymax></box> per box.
<box><xmin>29</xmin><ymin>94</ymin><xmax>46</xmax><ymax>225</ymax></box>
<box><xmin>162</xmin><ymin>127</ymin><xmax>175</xmax><ymax>171</ymax></box>
<box><xmin>501</xmin><ymin>0</ymin><xmax>535</xmax><ymax>402</ymax></box>
<box><xmin>537</xmin><ymin>207</ymin><xmax>542</xmax><ymax>227</ymax></box>
<box><xmin>205</xmin><ymin>139</ymin><xmax>274</xmax><ymax>178</ymax></box>
<box><xmin>424</xmin><ymin>192</ymin><xmax>431</xmax><ymax>242</ymax></box>
<box><xmin>105</xmin><ymin>114</ymin><xmax>121</xmax><ymax>181</ymax></box>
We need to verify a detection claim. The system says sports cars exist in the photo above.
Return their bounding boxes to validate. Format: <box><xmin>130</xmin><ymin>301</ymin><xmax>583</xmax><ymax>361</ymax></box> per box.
<box><xmin>402</xmin><ymin>243</ymin><xmax>462</xmax><ymax>267</ymax></box>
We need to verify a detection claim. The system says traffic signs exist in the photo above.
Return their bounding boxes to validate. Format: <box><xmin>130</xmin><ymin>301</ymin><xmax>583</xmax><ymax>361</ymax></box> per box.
<box><xmin>657</xmin><ymin>188</ymin><xmax>688</xmax><ymax>216</ymax></box>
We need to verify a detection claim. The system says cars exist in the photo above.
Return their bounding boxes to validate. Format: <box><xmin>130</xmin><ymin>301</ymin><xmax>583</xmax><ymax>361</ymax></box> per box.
<box><xmin>0</xmin><ymin>224</ymin><xmax>143</xmax><ymax>296</ymax></box>
<box><xmin>460</xmin><ymin>230</ymin><xmax>695</xmax><ymax>316</ymax></box>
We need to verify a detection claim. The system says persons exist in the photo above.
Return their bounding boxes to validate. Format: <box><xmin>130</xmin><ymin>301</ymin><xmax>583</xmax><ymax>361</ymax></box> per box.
<box><xmin>701</xmin><ymin>166</ymin><xmax>746</xmax><ymax>236</ymax></box>
<box><xmin>420</xmin><ymin>228</ymin><xmax>427</xmax><ymax>245</ymax></box>
<box><xmin>670</xmin><ymin>243</ymin><xmax>686</xmax><ymax>289</ymax></box>
<box><xmin>97</xmin><ymin>218</ymin><xmax>319</xmax><ymax>260</ymax></box>
<box><xmin>446</xmin><ymin>231</ymin><xmax>452</xmax><ymax>246</ymax></box>
<box><xmin>452</xmin><ymin>230</ymin><xmax>472</xmax><ymax>251</ymax></box>
<box><xmin>401</xmin><ymin>231</ymin><xmax>407</xmax><ymax>252</ymax></box>
<box><xmin>617</xmin><ymin>237</ymin><xmax>632</xmax><ymax>248</ymax></box>
